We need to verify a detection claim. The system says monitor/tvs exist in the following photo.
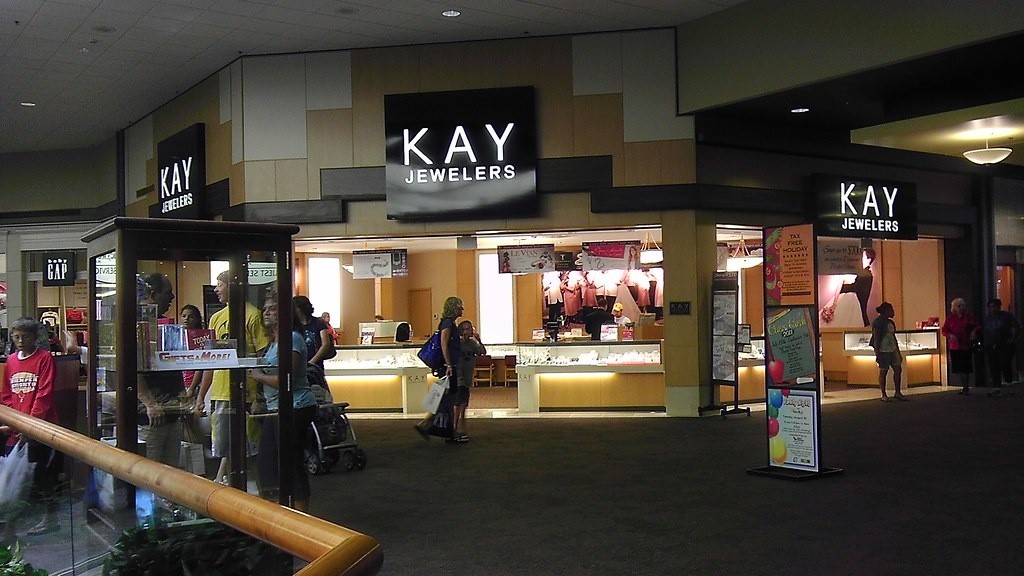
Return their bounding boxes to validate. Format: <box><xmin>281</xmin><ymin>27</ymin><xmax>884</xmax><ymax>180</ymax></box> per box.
<box><xmin>638</xmin><ymin>313</ymin><xmax>656</xmax><ymax>325</ymax></box>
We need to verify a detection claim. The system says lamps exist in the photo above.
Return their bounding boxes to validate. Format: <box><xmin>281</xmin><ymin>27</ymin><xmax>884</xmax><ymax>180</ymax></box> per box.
<box><xmin>962</xmin><ymin>140</ymin><xmax>1012</xmax><ymax>167</ymax></box>
<box><xmin>728</xmin><ymin>235</ymin><xmax>763</xmax><ymax>269</ymax></box>
<box><xmin>343</xmin><ymin>265</ymin><xmax>355</xmax><ymax>275</ymax></box>
<box><xmin>639</xmin><ymin>231</ymin><xmax>663</xmax><ymax>265</ymax></box>
<box><xmin>457</xmin><ymin>234</ymin><xmax>478</xmax><ymax>250</ymax></box>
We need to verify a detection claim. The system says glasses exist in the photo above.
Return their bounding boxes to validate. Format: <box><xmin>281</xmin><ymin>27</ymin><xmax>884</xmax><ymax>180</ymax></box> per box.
<box><xmin>955</xmin><ymin>304</ymin><xmax>963</xmax><ymax>307</ymax></box>
<box><xmin>462</xmin><ymin>326</ymin><xmax>475</xmax><ymax>330</ymax></box>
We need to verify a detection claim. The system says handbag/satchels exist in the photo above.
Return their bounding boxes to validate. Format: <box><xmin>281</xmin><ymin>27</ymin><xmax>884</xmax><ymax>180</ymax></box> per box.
<box><xmin>970</xmin><ymin>334</ymin><xmax>983</xmax><ymax>352</ymax></box>
<box><xmin>418</xmin><ymin>317</ymin><xmax>452</xmax><ymax>369</ymax></box>
<box><xmin>181</xmin><ymin>416</ymin><xmax>206</xmax><ymax>475</ymax></box>
<box><xmin>424</xmin><ymin>375</ymin><xmax>449</xmax><ymax>414</ymax></box>
<box><xmin>428</xmin><ymin>377</ymin><xmax>456</xmax><ymax>439</ymax></box>
<box><xmin>313</xmin><ymin>318</ymin><xmax>336</xmax><ymax>359</ymax></box>
<box><xmin>0</xmin><ymin>440</ymin><xmax>37</xmax><ymax>524</ymax></box>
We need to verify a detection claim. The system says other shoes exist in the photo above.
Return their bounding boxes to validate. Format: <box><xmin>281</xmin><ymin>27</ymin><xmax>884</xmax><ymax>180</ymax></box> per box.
<box><xmin>155</xmin><ymin>506</ymin><xmax>184</xmax><ymax>523</ymax></box>
<box><xmin>415</xmin><ymin>423</ymin><xmax>430</xmax><ymax>440</ymax></box>
<box><xmin>1007</xmin><ymin>383</ymin><xmax>1016</xmax><ymax>395</ymax></box>
<box><xmin>294</xmin><ymin>498</ymin><xmax>308</xmax><ymax>512</ymax></box>
<box><xmin>445</xmin><ymin>433</ymin><xmax>470</xmax><ymax>445</ymax></box>
<box><xmin>994</xmin><ymin>387</ymin><xmax>1000</xmax><ymax>397</ymax></box>
<box><xmin>958</xmin><ymin>387</ymin><xmax>969</xmax><ymax>395</ymax></box>
<box><xmin>222</xmin><ymin>475</ymin><xmax>230</xmax><ymax>486</ymax></box>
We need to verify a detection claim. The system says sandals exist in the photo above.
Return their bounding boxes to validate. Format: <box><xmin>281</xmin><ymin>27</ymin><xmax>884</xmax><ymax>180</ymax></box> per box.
<box><xmin>880</xmin><ymin>396</ymin><xmax>892</xmax><ymax>402</ymax></box>
<box><xmin>894</xmin><ymin>394</ymin><xmax>910</xmax><ymax>401</ymax></box>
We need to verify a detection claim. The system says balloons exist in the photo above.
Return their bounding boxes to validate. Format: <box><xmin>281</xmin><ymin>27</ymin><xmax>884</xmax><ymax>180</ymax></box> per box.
<box><xmin>769</xmin><ymin>389</ymin><xmax>790</xmax><ymax>463</ymax></box>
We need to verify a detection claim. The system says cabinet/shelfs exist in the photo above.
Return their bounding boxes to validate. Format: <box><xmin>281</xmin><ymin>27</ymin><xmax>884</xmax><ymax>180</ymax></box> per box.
<box><xmin>715</xmin><ymin>327</ymin><xmax>943</xmax><ymax>405</ymax></box>
<box><xmin>323</xmin><ymin>328</ymin><xmax>666</xmax><ymax>410</ymax></box>
<box><xmin>79</xmin><ymin>215</ymin><xmax>301</xmax><ymax>576</ymax></box>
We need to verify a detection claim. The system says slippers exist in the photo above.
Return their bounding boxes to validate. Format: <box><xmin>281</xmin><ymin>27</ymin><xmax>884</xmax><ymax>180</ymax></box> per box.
<box><xmin>28</xmin><ymin>521</ymin><xmax>60</xmax><ymax>536</ymax></box>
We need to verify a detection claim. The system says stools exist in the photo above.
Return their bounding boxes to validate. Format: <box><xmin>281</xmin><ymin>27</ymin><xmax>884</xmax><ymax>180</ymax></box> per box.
<box><xmin>473</xmin><ymin>355</ymin><xmax>496</xmax><ymax>389</ymax></box>
<box><xmin>505</xmin><ymin>356</ymin><xmax>517</xmax><ymax>388</ymax></box>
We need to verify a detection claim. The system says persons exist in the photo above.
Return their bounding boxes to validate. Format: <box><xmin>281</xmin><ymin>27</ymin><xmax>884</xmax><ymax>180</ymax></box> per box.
<box><xmin>819</xmin><ymin>249</ymin><xmax>876</xmax><ymax>327</ymax></box>
<box><xmin>980</xmin><ymin>298</ymin><xmax>1021</xmax><ymax>398</ymax></box>
<box><xmin>942</xmin><ymin>298</ymin><xmax>982</xmax><ymax>396</ymax></box>
<box><xmin>137</xmin><ymin>273</ymin><xmax>188</xmax><ymax>506</ymax></box>
<box><xmin>613</xmin><ymin>303</ymin><xmax>631</xmax><ymax>325</ymax></box>
<box><xmin>181</xmin><ymin>304</ymin><xmax>204</xmax><ymax>443</ymax></box>
<box><xmin>414</xmin><ymin>297</ymin><xmax>486</xmax><ymax>443</ymax></box>
<box><xmin>627</xmin><ymin>246</ymin><xmax>639</xmax><ymax>269</ymax></box>
<box><xmin>872</xmin><ymin>301</ymin><xmax>911</xmax><ymax>401</ymax></box>
<box><xmin>194</xmin><ymin>270</ymin><xmax>273</xmax><ymax>497</ymax></box>
<box><xmin>0</xmin><ymin>317</ymin><xmax>61</xmax><ymax>546</ymax></box>
<box><xmin>294</xmin><ymin>296</ymin><xmax>330</xmax><ymax>374</ymax></box>
<box><xmin>585</xmin><ymin>298</ymin><xmax>614</xmax><ymax>341</ymax></box>
<box><xmin>248</xmin><ymin>295</ymin><xmax>317</xmax><ymax>513</ymax></box>
<box><xmin>321</xmin><ymin>312</ymin><xmax>340</xmax><ymax>345</ymax></box>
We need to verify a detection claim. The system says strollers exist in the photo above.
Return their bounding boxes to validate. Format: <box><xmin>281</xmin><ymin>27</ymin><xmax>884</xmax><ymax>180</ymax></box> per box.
<box><xmin>303</xmin><ymin>363</ymin><xmax>368</xmax><ymax>475</ymax></box>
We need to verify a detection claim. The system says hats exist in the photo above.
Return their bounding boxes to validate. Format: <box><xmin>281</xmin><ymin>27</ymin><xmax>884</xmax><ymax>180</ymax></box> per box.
<box><xmin>613</xmin><ymin>303</ymin><xmax>623</xmax><ymax>311</ymax></box>
